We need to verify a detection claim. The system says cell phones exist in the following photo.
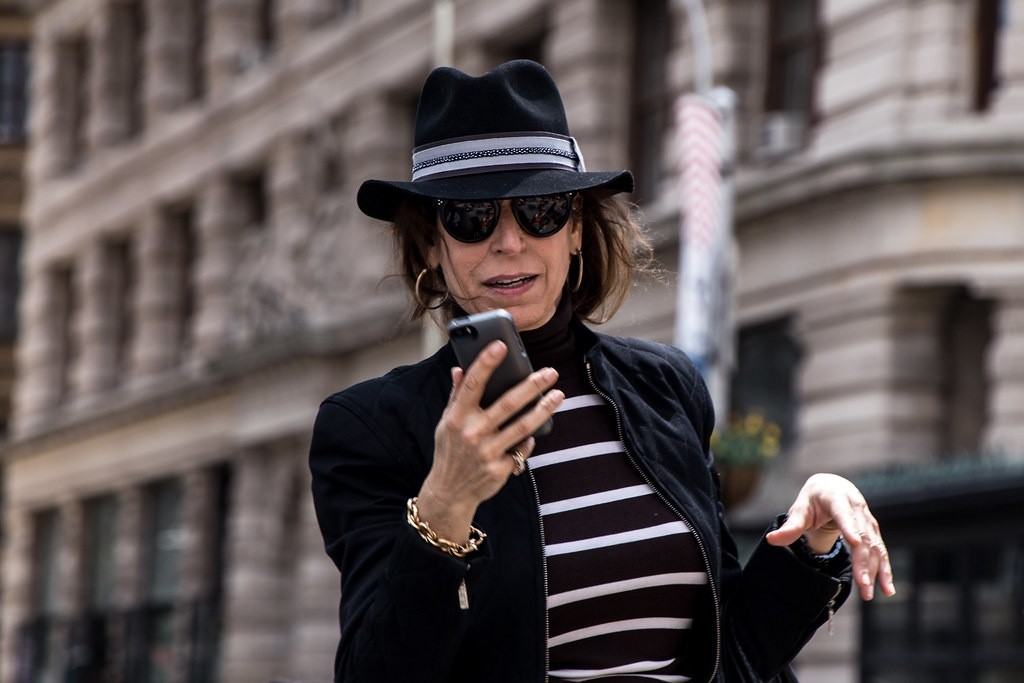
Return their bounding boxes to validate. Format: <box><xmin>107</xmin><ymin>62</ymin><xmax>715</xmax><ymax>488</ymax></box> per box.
<box><xmin>449</xmin><ymin>310</ymin><xmax>555</xmax><ymax>437</ymax></box>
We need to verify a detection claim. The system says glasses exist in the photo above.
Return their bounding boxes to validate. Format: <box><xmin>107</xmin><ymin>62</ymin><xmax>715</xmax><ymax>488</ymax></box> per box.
<box><xmin>434</xmin><ymin>192</ymin><xmax>577</xmax><ymax>243</ymax></box>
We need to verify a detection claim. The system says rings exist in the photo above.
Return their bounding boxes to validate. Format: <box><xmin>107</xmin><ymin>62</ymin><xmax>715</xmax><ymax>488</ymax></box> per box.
<box><xmin>510</xmin><ymin>448</ymin><xmax>526</xmax><ymax>477</ymax></box>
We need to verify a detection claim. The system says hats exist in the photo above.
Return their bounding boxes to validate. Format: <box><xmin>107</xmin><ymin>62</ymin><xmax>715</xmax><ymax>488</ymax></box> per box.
<box><xmin>357</xmin><ymin>60</ymin><xmax>634</xmax><ymax>222</ymax></box>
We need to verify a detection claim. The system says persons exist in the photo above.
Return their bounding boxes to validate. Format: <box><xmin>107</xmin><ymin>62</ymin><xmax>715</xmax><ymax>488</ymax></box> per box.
<box><xmin>308</xmin><ymin>59</ymin><xmax>897</xmax><ymax>683</ymax></box>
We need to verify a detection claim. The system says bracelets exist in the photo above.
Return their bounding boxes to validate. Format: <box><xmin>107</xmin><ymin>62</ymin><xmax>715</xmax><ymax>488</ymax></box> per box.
<box><xmin>812</xmin><ymin>534</ymin><xmax>844</xmax><ymax>565</ymax></box>
<box><xmin>406</xmin><ymin>496</ymin><xmax>487</xmax><ymax>559</ymax></box>
